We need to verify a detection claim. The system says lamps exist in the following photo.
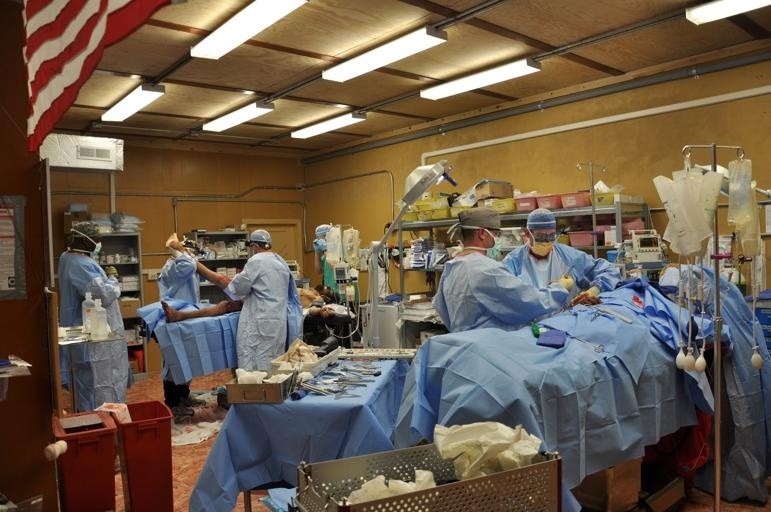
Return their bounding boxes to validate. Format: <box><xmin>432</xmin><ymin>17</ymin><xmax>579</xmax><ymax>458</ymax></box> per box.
<box><xmin>101</xmin><ymin>83</ymin><xmax>165</xmax><ymax>123</ymax></box>
<box><xmin>686</xmin><ymin>1</ymin><xmax>771</xmax><ymax>27</ymax></box>
<box><xmin>419</xmin><ymin>57</ymin><xmax>542</xmax><ymax>101</ymax></box>
<box><xmin>322</xmin><ymin>25</ymin><xmax>448</xmax><ymax>84</ymax></box>
<box><xmin>202</xmin><ymin>100</ymin><xmax>274</xmax><ymax>132</ymax></box>
<box><xmin>188</xmin><ymin>0</ymin><xmax>310</xmax><ymax>60</ymax></box>
<box><xmin>290</xmin><ymin>112</ymin><xmax>367</xmax><ymax>140</ymax></box>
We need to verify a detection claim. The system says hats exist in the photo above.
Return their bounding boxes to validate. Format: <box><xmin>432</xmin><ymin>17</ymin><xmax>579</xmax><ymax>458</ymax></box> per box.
<box><xmin>458</xmin><ymin>208</ymin><xmax>501</xmax><ymax>230</ymax></box>
<box><xmin>72</xmin><ymin>221</ymin><xmax>100</xmax><ymax>238</ymax></box>
<box><xmin>315</xmin><ymin>224</ymin><xmax>332</xmax><ymax>238</ymax></box>
<box><xmin>527</xmin><ymin>207</ymin><xmax>557</xmax><ymax>230</ymax></box>
<box><xmin>251</xmin><ymin>229</ymin><xmax>273</xmax><ymax>243</ymax></box>
<box><xmin>182</xmin><ymin>240</ymin><xmax>200</xmax><ymax>254</ymax></box>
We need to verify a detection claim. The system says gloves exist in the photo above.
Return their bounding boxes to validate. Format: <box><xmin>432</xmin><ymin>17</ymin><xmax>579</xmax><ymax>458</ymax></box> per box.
<box><xmin>168</xmin><ymin>247</ymin><xmax>182</xmax><ymax>260</ymax></box>
<box><xmin>559</xmin><ymin>274</ymin><xmax>574</xmax><ymax>291</ymax></box>
<box><xmin>106</xmin><ymin>266</ymin><xmax>118</xmax><ymax>278</ymax></box>
<box><xmin>572</xmin><ymin>286</ymin><xmax>601</xmax><ymax>307</ymax></box>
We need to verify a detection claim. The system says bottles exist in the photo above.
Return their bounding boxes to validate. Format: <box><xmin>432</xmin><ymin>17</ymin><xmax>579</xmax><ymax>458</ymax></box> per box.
<box><xmin>80</xmin><ymin>290</ymin><xmax>109</xmax><ymax>341</ymax></box>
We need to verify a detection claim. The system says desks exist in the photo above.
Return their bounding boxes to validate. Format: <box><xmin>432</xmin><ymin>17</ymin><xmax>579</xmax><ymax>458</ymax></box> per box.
<box><xmin>188</xmin><ymin>358</ymin><xmax>408</xmax><ymax>511</ymax></box>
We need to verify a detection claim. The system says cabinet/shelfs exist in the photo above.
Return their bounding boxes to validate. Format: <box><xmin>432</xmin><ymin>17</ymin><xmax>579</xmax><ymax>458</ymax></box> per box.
<box><xmin>360</xmin><ymin>304</ymin><xmax>398</xmax><ymax>348</ymax></box>
<box><xmin>398</xmin><ymin>195</ymin><xmax>648</xmax><ymax>349</ymax></box>
<box><xmin>191</xmin><ymin>229</ymin><xmax>250</xmax><ymax>304</ymax></box>
<box><xmin>67</xmin><ymin>231</ymin><xmax>148</xmax><ymax>381</ymax></box>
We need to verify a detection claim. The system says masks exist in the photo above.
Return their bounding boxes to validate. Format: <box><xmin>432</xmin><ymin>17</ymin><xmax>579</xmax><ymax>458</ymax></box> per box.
<box><xmin>187</xmin><ymin>250</ymin><xmax>198</xmax><ymax>261</ymax></box>
<box><xmin>525</xmin><ymin>226</ymin><xmax>557</xmax><ymax>257</ymax></box>
<box><xmin>318</xmin><ymin>239</ymin><xmax>327</xmax><ymax>251</ymax></box>
<box><xmin>478</xmin><ymin>227</ymin><xmax>501</xmax><ymax>258</ymax></box>
<box><xmin>83</xmin><ymin>235</ymin><xmax>102</xmax><ymax>258</ymax></box>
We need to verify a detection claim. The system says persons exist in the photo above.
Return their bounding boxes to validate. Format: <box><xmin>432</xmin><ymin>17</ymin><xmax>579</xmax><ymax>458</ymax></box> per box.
<box><xmin>312</xmin><ymin>224</ymin><xmax>359</xmax><ymax>305</ymax></box>
<box><xmin>499</xmin><ymin>207</ymin><xmax>622</xmax><ymax>309</ymax></box>
<box><xmin>156</xmin><ymin>238</ymin><xmax>207</xmax><ymax>416</ymax></box>
<box><xmin>160</xmin><ymin>232</ymin><xmax>341</xmax><ymax>323</ymax></box>
<box><xmin>432</xmin><ymin>207</ymin><xmax>575</xmax><ymax>333</ymax></box>
<box><xmin>57</xmin><ymin>219</ymin><xmax>137</xmax><ymax>413</ymax></box>
<box><xmin>227</xmin><ymin>229</ymin><xmax>304</xmax><ymax>372</ymax></box>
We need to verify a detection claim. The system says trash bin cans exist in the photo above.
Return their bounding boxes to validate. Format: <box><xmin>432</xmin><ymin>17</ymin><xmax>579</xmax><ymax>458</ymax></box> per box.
<box><xmin>53</xmin><ymin>400</ymin><xmax>174</xmax><ymax>512</ymax></box>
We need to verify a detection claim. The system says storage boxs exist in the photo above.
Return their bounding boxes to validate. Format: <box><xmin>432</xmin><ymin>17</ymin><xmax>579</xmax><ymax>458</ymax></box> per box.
<box><xmin>227</xmin><ymin>370</ymin><xmax>297</xmax><ymax>405</ymax></box>
<box><xmin>63</xmin><ymin>212</ymin><xmax>87</xmax><ymax>235</ymax></box>
<box><xmin>118</xmin><ymin>296</ymin><xmax>141</xmax><ymax>317</ymax></box>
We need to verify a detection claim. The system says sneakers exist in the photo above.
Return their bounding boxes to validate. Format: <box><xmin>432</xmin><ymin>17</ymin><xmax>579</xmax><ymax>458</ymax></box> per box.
<box><xmin>170</xmin><ymin>405</ymin><xmax>195</xmax><ymax>417</ymax></box>
<box><xmin>179</xmin><ymin>392</ymin><xmax>207</xmax><ymax>408</ymax></box>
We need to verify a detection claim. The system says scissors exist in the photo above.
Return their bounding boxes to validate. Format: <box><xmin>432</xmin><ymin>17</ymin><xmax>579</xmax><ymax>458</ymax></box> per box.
<box><xmin>334</xmin><ymin>392</ymin><xmax>361</xmax><ymax>400</ymax></box>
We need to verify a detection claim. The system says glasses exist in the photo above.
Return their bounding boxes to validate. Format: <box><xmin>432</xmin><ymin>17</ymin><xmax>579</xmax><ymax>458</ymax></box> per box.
<box><xmin>489</xmin><ymin>228</ymin><xmax>502</xmax><ymax>238</ymax></box>
<box><xmin>530</xmin><ymin>230</ymin><xmax>558</xmax><ymax>242</ymax></box>
